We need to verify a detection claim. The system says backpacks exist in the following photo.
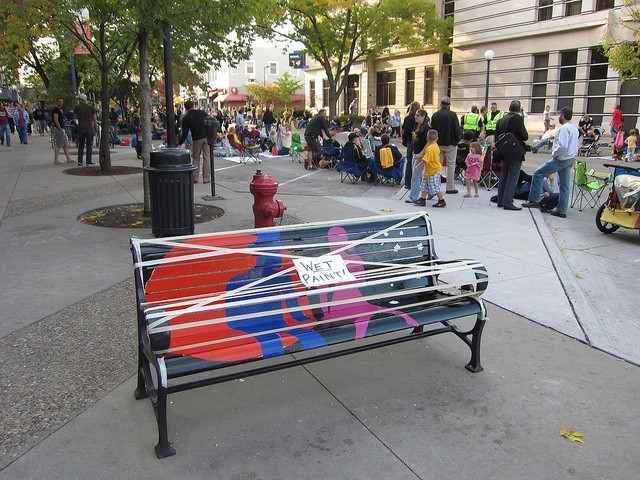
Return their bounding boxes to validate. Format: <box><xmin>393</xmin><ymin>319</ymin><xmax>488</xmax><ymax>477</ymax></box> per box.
<box><xmin>540</xmin><ymin>193</ymin><xmax>559</xmax><ymax>213</ymax></box>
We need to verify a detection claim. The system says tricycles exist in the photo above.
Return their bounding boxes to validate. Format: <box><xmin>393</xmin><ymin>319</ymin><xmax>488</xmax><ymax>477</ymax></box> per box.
<box><xmin>51</xmin><ymin>117</ymin><xmax>74</xmax><ymax>148</ymax></box>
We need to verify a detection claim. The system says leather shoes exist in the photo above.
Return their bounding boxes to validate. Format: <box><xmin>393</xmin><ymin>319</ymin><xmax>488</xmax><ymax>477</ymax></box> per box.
<box><xmin>307</xmin><ymin>165</ymin><xmax>317</xmax><ymax>170</ymax></box>
<box><xmin>203</xmin><ymin>181</ymin><xmax>210</xmax><ymax>184</ymax></box>
<box><xmin>522</xmin><ymin>201</ymin><xmax>539</xmax><ymax>208</ymax></box>
<box><xmin>504</xmin><ymin>205</ymin><xmax>522</xmax><ymax>210</ymax></box>
<box><xmin>498</xmin><ymin>204</ymin><xmax>504</xmax><ymax>207</ymax></box>
<box><xmin>446</xmin><ymin>190</ymin><xmax>458</xmax><ymax>194</ymax></box>
<box><xmin>194</xmin><ymin>180</ymin><xmax>198</xmax><ymax>183</ymax></box>
<box><xmin>551</xmin><ymin>211</ymin><xmax>566</xmax><ymax>218</ymax></box>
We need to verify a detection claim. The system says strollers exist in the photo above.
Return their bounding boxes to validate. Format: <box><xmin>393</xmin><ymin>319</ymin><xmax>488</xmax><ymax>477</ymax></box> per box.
<box><xmin>579</xmin><ymin>125</ymin><xmax>605</xmax><ymax>158</ymax></box>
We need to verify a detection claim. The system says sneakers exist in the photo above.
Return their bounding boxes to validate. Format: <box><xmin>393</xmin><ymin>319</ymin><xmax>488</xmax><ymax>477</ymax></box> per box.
<box><xmin>67</xmin><ymin>158</ymin><xmax>76</xmax><ymax>163</ymax></box>
<box><xmin>55</xmin><ymin>160</ymin><xmax>64</xmax><ymax>164</ymax></box>
<box><xmin>427</xmin><ymin>193</ymin><xmax>436</xmax><ymax>200</ymax></box>
<box><xmin>87</xmin><ymin>162</ymin><xmax>97</xmax><ymax>166</ymax></box>
<box><xmin>78</xmin><ymin>162</ymin><xmax>83</xmax><ymax>166</ymax></box>
<box><xmin>405</xmin><ymin>197</ymin><xmax>417</xmax><ymax>203</ymax></box>
<box><xmin>432</xmin><ymin>199</ymin><xmax>446</xmax><ymax>207</ymax></box>
<box><xmin>413</xmin><ymin>198</ymin><xmax>426</xmax><ymax>206</ymax></box>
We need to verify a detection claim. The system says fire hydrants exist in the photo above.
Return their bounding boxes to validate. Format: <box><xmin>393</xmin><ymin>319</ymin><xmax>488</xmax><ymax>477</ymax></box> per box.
<box><xmin>250</xmin><ymin>170</ymin><xmax>286</xmax><ymax>269</ymax></box>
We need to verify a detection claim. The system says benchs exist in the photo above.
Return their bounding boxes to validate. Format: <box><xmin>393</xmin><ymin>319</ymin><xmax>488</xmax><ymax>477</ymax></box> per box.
<box><xmin>128</xmin><ymin>209</ymin><xmax>489</xmax><ymax>461</ymax></box>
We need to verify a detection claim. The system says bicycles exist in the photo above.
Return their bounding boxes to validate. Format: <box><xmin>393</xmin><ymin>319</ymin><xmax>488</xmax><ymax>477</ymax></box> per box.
<box><xmin>109</xmin><ymin>124</ymin><xmax>116</xmax><ymax>149</ymax></box>
<box><xmin>92</xmin><ymin>119</ymin><xmax>102</xmax><ymax>149</ymax></box>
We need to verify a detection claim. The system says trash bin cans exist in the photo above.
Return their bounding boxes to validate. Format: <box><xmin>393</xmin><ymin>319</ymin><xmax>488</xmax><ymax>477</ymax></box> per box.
<box><xmin>144</xmin><ymin>144</ymin><xmax>199</xmax><ymax>237</ymax></box>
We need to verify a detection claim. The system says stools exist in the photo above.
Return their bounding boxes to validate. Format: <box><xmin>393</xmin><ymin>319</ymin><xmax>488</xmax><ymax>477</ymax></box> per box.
<box><xmin>338</xmin><ymin>162</ymin><xmax>370</xmax><ymax>185</ymax></box>
<box><xmin>311</xmin><ymin>145</ymin><xmax>341</xmax><ymax>170</ymax></box>
<box><xmin>371</xmin><ymin>158</ymin><xmax>406</xmax><ymax>186</ymax></box>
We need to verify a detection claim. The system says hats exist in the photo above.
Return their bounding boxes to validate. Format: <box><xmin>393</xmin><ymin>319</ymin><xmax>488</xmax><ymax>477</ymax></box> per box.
<box><xmin>353</xmin><ymin>127</ymin><xmax>360</xmax><ymax>133</ymax></box>
<box><xmin>360</xmin><ymin>128</ymin><xmax>368</xmax><ymax>134</ymax></box>
<box><xmin>348</xmin><ymin>133</ymin><xmax>359</xmax><ymax>138</ymax></box>
<box><xmin>75</xmin><ymin>94</ymin><xmax>87</xmax><ymax>101</ymax></box>
<box><xmin>441</xmin><ymin>96</ymin><xmax>451</xmax><ymax>105</ymax></box>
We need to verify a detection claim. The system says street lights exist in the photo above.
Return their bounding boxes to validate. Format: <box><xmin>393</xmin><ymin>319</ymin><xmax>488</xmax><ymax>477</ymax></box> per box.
<box><xmin>263</xmin><ymin>64</ymin><xmax>269</xmax><ymax>86</ymax></box>
<box><xmin>483</xmin><ymin>50</ymin><xmax>494</xmax><ymax>112</ymax></box>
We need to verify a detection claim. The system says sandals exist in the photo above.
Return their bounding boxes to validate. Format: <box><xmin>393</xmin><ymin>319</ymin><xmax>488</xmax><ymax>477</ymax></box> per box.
<box><xmin>474</xmin><ymin>194</ymin><xmax>479</xmax><ymax>197</ymax></box>
<box><xmin>464</xmin><ymin>193</ymin><xmax>471</xmax><ymax>197</ymax></box>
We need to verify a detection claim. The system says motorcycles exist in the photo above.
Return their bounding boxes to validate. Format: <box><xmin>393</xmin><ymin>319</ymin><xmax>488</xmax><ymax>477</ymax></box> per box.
<box><xmin>596</xmin><ymin>159</ymin><xmax>640</xmax><ymax>237</ymax></box>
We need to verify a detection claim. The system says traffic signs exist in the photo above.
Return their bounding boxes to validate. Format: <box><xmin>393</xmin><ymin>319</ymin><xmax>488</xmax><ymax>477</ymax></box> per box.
<box><xmin>294</xmin><ymin>50</ymin><xmax>305</xmax><ymax>68</ymax></box>
<box><xmin>288</xmin><ymin>53</ymin><xmax>303</xmax><ymax>68</ymax></box>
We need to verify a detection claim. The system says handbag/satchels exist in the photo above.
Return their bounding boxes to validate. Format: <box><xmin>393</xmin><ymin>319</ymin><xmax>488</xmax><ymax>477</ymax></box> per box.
<box><xmin>495</xmin><ymin>114</ymin><xmax>527</xmax><ymax>163</ymax></box>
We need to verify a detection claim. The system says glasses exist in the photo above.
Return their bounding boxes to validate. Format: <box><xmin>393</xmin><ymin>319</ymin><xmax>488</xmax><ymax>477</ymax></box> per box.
<box><xmin>414</xmin><ymin>113</ymin><xmax>422</xmax><ymax>117</ymax></box>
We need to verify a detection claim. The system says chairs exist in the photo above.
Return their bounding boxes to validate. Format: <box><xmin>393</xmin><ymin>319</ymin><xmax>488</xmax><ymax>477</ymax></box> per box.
<box><xmin>237</xmin><ymin>130</ymin><xmax>259</xmax><ymax>165</ymax></box>
<box><xmin>288</xmin><ymin>133</ymin><xmax>309</xmax><ymax>163</ymax></box>
<box><xmin>571</xmin><ymin>159</ymin><xmax>602</xmax><ymax>211</ymax></box>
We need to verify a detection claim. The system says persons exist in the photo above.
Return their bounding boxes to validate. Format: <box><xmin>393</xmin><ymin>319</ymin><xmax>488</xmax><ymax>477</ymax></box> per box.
<box><xmin>482</xmin><ymin>103</ymin><xmax>505</xmax><ymax>140</ymax></box>
<box><xmin>614</xmin><ymin>124</ymin><xmax>624</xmax><ymax>161</ymax></box>
<box><xmin>464</xmin><ymin>142</ymin><xmax>484</xmax><ymax>197</ymax></box>
<box><xmin>431</xmin><ymin>97</ymin><xmax>458</xmax><ymax>193</ymax></box>
<box><xmin>51</xmin><ymin>97</ymin><xmax>76</xmax><ymax>165</ymax></box>
<box><xmin>296</xmin><ymin>110</ymin><xmax>309</xmax><ymax>129</ymax></box>
<box><xmin>539</xmin><ymin>172</ymin><xmax>560</xmax><ymax>213</ymax></box>
<box><xmin>610</xmin><ymin>104</ymin><xmax>627</xmax><ymax>142</ymax></box>
<box><xmin>461</xmin><ymin>105</ymin><xmax>483</xmax><ymax>142</ymax></box>
<box><xmin>72</xmin><ymin>93</ymin><xmax>100</xmax><ymax>166</ymax></box>
<box><xmin>304</xmin><ymin>109</ymin><xmax>334</xmax><ymax>169</ymax></box>
<box><xmin>177</xmin><ymin>102</ymin><xmax>211</xmax><ymax>183</ymax></box>
<box><xmin>413</xmin><ymin>129</ymin><xmax>446</xmax><ymax>207</ymax></box>
<box><xmin>514</xmin><ymin>170</ymin><xmax>533</xmax><ymax>199</ymax></box>
<box><xmin>496</xmin><ymin>100</ymin><xmax>528</xmax><ymax>210</ymax></box>
<box><xmin>338</xmin><ymin>117</ymin><xmax>353</xmax><ymax>131</ymax></box>
<box><xmin>633</xmin><ymin>128</ymin><xmax>640</xmax><ymax>152</ymax></box>
<box><xmin>361</xmin><ymin>105</ymin><xmax>400</xmax><ymax>133</ymax></box>
<box><xmin>353</xmin><ymin>128</ymin><xmax>362</xmax><ymax>152</ymax></box>
<box><xmin>324</xmin><ymin>132</ymin><xmax>343</xmax><ymax>155</ymax></box>
<box><xmin>211</xmin><ymin>104</ymin><xmax>296</xmax><ymax>155</ymax></box>
<box><xmin>119</xmin><ymin>105</ymin><xmax>176</xmax><ymax>145</ymax></box>
<box><xmin>365</xmin><ymin>128</ymin><xmax>375</xmax><ymax>152</ymax></box>
<box><xmin>521</xmin><ymin>106</ymin><xmax>579</xmax><ymax>218</ymax></box>
<box><xmin>578</xmin><ymin>114</ymin><xmax>593</xmax><ymax>138</ymax></box>
<box><xmin>478</xmin><ymin>106</ymin><xmax>488</xmax><ymax>140</ymax></box>
<box><xmin>543</xmin><ymin>105</ymin><xmax>554</xmax><ymax>134</ymax></box>
<box><xmin>343</xmin><ymin>133</ymin><xmax>377</xmax><ymax>182</ymax></box>
<box><xmin>459</xmin><ymin>130</ymin><xmax>475</xmax><ymax>146</ymax></box>
<box><xmin>0</xmin><ymin>99</ymin><xmax>50</xmax><ymax>147</ymax></box>
<box><xmin>530</xmin><ymin>120</ymin><xmax>558</xmax><ymax>153</ymax></box>
<box><xmin>405</xmin><ymin>109</ymin><xmax>431</xmax><ymax>203</ymax></box>
<box><xmin>375</xmin><ymin>133</ymin><xmax>403</xmax><ymax>180</ymax></box>
<box><xmin>581</xmin><ymin>129</ymin><xmax>598</xmax><ymax>146</ymax></box>
<box><xmin>328</xmin><ymin>115</ymin><xmax>341</xmax><ymax>130</ymax></box>
<box><xmin>483</xmin><ymin>135</ymin><xmax>495</xmax><ymax>158</ymax></box>
<box><xmin>108</xmin><ymin>106</ymin><xmax>118</xmax><ymax>136</ymax></box>
<box><xmin>624</xmin><ymin>128</ymin><xmax>637</xmax><ymax>161</ymax></box>
<box><xmin>402</xmin><ymin>101</ymin><xmax>429</xmax><ymax>189</ymax></box>
